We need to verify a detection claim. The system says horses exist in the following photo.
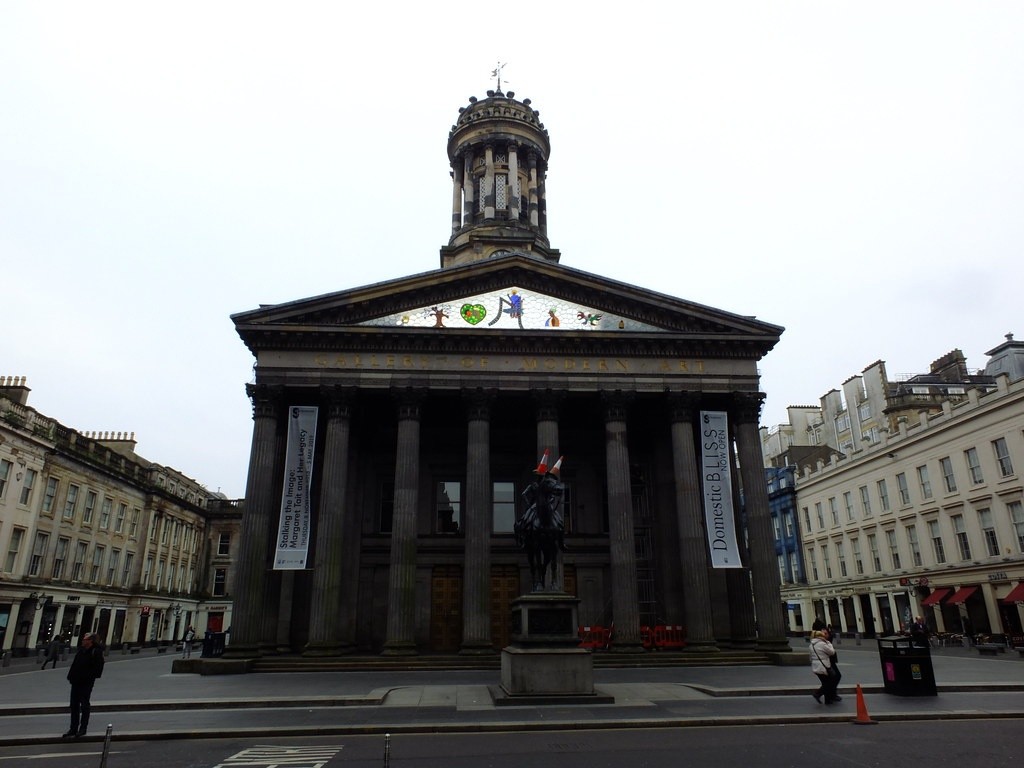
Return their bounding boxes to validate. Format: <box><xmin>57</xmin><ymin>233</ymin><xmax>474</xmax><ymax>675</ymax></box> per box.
<box><xmin>515</xmin><ymin>471</ymin><xmax>569</xmax><ymax>594</ymax></box>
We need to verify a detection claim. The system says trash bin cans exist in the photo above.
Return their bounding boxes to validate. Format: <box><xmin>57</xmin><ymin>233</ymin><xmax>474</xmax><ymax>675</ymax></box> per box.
<box><xmin>877</xmin><ymin>635</ymin><xmax>939</xmax><ymax>697</ymax></box>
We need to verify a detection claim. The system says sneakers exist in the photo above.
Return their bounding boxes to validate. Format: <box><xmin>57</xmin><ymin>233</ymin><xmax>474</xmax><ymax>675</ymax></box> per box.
<box><xmin>62</xmin><ymin>728</ymin><xmax>78</xmax><ymax>737</ymax></box>
<box><xmin>812</xmin><ymin>693</ymin><xmax>823</xmax><ymax>704</ymax></box>
<box><xmin>833</xmin><ymin>694</ymin><xmax>842</xmax><ymax>701</ymax></box>
<box><xmin>825</xmin><ymin>701</ymin><xmax>833</xmax><ymax>705</ymax></box>
<box><xmin>75</xmin><ymin>729</ymin><xmax>86</xmax><ymax>737</ymax></box>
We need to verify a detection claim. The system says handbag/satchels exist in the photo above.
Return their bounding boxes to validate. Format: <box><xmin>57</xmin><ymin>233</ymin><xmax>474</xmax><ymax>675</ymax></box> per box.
<box><xmin>825</xmin><ymin>662</ymin><xmax>842</xmax><ymax>685</ymax></box>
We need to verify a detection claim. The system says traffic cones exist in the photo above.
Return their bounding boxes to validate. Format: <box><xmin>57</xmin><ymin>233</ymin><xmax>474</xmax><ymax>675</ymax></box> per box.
<box><xmin>850</xmin><ymin>684</ymin><xmax>878</xmax><ymax>726</ymax></box>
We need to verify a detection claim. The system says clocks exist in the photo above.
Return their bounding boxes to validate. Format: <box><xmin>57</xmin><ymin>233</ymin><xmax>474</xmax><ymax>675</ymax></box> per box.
<box><xmin>489</xmin><ymin>250</ymin><xmax>511</xmax><ymax>259</ymax></box>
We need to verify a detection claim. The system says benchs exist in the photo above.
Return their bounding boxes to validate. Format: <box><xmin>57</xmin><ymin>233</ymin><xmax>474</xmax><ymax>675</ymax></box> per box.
<box><xmin>931</xmin><ymin>636</ymin><xmax>1024</xmax><ymax>653</ymax></box>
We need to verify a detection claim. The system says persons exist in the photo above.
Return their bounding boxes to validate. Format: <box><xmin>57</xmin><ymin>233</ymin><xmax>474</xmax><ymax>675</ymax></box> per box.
<box><xmin>513</xmin><ymin>469</ymin><xmax>567</xmax><ymax>553</ymax></box>
<box><xmin>809</xmin><ymin>630</ymin><xmax>835</xmax><ymax>705</ymax></box>
<box><xmin>181</xmin><ymin>625</ymin><xmax>195</xmax><ymax>659</ymax></box>
<box><xmin>820</xmin><ymin>628</ymin><xmax>842</xmax><ymax>701</ymax></box>
<box><xmin>41</xmin><ymin>634</ymin><xmax>61</xmax><ymax>670</ymax></box>
<box><xmin>910</xmin><ymin>616</ymin><xmax>930</xmax><ymax>649</ymax></box>
<box><xmin>63</xmin><ymin>632</ymin><xmax>104</xmax><ymax>737</ymax></box>
<box><xmin>812</xmin><ymin>618</ymin><xmax>825</xmax><ymax>631</ymax></box>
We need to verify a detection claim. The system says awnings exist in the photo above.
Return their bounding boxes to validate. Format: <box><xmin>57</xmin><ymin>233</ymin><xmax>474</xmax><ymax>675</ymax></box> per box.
<box><xmin>947</xmin><ymin>587</ymin><xmax>977</xmax><ymax>604</ymax></box>
<box><xmin>1003</xmin><ymin>582</ymin><xmax>1024</xmax><ymax>602</ymax></box>
<box><xmin>920</xmin><ymin>588</ymin><xmax>951</xmax><ymax>606</ymax></box>
